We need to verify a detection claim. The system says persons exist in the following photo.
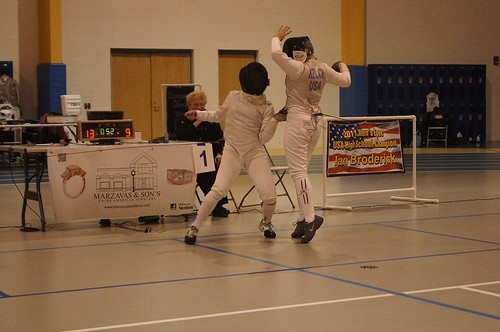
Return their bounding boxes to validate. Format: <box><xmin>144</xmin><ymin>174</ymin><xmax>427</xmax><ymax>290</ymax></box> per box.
<box><xmin>0</xmin><ymin>73</ymin><xmax>21</xmax><ymax>107</ymax></box>
<box><xmin>175</xmin><ymin>91</ymin><xmax>230</xmax><ymax>217</ymax></box>
<box><xmin>270</xmin><ymin>25</ymin><xmax>351</xmax><ymax>244</ymax></box>
<box><xmin>183</xmin><ymin>62</ymin><xmax>286</xmax><ymax>245</ymax></box>
<box><xmin>420</xmin><ymin>107</ymin><xmax>446</xmax><ymax>146</ymax></box>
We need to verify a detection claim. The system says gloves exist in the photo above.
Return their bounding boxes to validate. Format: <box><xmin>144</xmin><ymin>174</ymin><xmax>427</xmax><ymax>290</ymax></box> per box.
<box><xmin>332</xmin><ymin>61</ymin><xmax>343</xmax><ymax>73</ymax></box>
<box><xmin>273</xmin><ymin>107</ymin><xmax>288</xmax><ymax>122</ymax></box>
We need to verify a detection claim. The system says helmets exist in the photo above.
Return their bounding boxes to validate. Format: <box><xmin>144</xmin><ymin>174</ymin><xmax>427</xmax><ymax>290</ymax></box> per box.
<box><xmin>283</xmin><ymin>35</ymin><xmax>315</xmax><ymax>63</ymax></box>
<box><xmin>239</xmin><ymin>62</ymin><xmax>271</xmax><ymax>95</ymax></box>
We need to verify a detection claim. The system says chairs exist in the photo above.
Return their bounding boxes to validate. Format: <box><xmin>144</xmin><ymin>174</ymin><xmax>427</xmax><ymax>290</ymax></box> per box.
<box><xmin>236</xmin><ymin>145</ymin><xmax>295</xmax><ymax>209</ymax></box>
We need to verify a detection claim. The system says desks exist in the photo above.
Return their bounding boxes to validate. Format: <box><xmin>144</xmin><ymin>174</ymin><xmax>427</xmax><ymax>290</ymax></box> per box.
<box><xmin>0</xmin><ymin>141</ymin><xmax>195</xmax><ymax>232</ymax></box>
<box><xmin>0</xmin><ymin>123</ymin><xmax>76</xmax><ymax>144</ymax></box>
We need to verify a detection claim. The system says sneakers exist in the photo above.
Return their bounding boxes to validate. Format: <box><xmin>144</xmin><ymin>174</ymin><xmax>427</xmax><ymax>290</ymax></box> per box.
<box><xmin>291</xmin><ymin>218</ymin><xmax>307</xmax><ymax>237</ymax></box>
<box><xmin>185</xmin><ymin>227</ymin><xmax>199</xmax><ymax>245</ymax></box>
<box><xmin>301</xmin><ymin>215</ymin><xmax>325</xmax><ymax>243</ymax></box>
<box><xmin>257</xmin><ymin>219</ymin><xmax>277</xmax><ymax>239</ymax></box>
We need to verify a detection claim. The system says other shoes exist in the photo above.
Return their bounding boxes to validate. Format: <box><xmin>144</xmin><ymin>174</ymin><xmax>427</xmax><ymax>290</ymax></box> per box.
<box><xmin>212</xmin><ymin>206</ymin><xmax>230</xmax><ymax>217</ymax></box>
